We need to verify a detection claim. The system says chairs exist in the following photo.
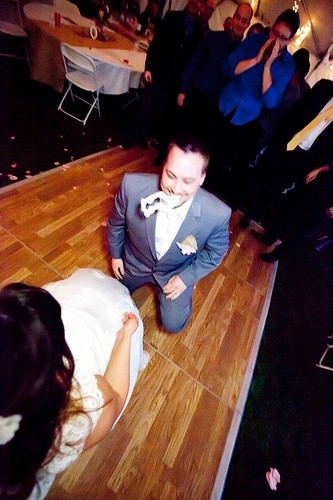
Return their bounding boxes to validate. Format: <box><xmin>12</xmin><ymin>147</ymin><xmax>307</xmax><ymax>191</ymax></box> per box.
<box><xmin>0</xmin><ymin>1</ymin><xmax>31</xmax><ymax>70</ymax></box>
<box><xmin>57</xmin><ymin>42</ymin><xmax>103</xmax><ymax>125</ymax></box>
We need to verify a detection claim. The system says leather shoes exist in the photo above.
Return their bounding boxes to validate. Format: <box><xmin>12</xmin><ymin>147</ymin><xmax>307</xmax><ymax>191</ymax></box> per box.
<box><xmin>240</xmin><ymin>215</ymin><xmax>250</xmax><ymax>228</ymax></box>
<box><xmin>228</xmin><ymin>203</ymin><xmax>238</xmax><ymax>212</ymax></box>
<box><xmin>260</xmin><ymin>252</ymin><xmax>276</xmax><ymax>262</ymax></box>
<box><xmin>251</xmin><ymin>228</ymin><xmax>272</xmax><ymax>247</ymax></box>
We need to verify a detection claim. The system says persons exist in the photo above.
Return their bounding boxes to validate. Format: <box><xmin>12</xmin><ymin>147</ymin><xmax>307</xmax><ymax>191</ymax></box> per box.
<box><xmin>1</xmin><ymin>268</ymin><xmax>150</xmax><ymax>500</ymax></box>
<box><xmin>250</xmin><ymin>157</ymin><xmax>333</xmax><ymax>263</ymax></box>
<box><xmin>122</xmin><ymin>0</ymin><xmax>311</xmax><ymax>191</ymax></box>
<box><xmin>228</xmin><ymin>79</ymin><xmax>333</xmax><ymax>229</ymax></box>
<box><xmin>107</xmin><ymin>131</ymin><xmax>233</xmax><ymax>333</ymax></box>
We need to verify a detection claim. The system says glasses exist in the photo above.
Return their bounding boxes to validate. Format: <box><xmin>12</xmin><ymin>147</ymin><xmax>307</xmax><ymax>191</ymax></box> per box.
<box><xmin>272</xmin><ymin>26</ymin><xmax>290</xmax><ymax>41</ymax></box>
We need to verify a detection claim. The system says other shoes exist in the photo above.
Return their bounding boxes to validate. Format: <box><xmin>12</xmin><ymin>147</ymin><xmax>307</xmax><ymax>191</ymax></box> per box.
<box><xmin>122</xmin><ymin>136</ymin><xmax>149</xmax><ymax>150</ymax></box>
<box><xmin>153</xmin><ymin>154</ymin><xmax>165</xmax><ymax>167</ymax></box>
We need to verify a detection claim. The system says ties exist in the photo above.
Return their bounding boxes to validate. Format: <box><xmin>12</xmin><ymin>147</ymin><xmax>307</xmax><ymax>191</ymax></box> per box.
<box><xmin>287</xmin><ymin>103</ymin><xmax>333</xmax><ymax>151</ymax></box>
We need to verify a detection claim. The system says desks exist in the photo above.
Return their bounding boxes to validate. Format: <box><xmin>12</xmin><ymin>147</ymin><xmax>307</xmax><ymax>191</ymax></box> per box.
<box><xmin>23</xmin><ymin>0</ymin><xmax>149</xmax><ymax>111</ymax></box>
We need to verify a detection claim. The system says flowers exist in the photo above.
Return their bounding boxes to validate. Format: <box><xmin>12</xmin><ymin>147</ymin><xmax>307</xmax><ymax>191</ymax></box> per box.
<box><xmin>265</xmin><ymin>467</ymin><xmax>281</xmax><ymax>491</ymax></box>
<box><xmin>176</xmin><ymin>236</ymin><xmax>198</xmax><ymax>255</ymax></box>
<box><xmin>0</xmin><ymin>415</ymin><xmax>22</xmax><ymax>445</ymax></box>
